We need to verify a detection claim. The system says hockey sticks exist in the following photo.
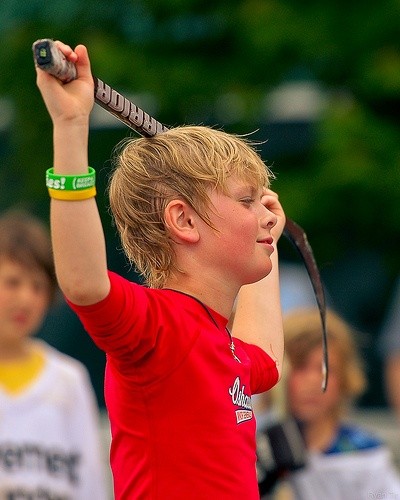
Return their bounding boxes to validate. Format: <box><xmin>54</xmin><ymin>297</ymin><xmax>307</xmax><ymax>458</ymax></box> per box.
<box><xmin>32</xmin><ymin>38</ymin><xmax>330</xmax><ymax>392</ymax></box>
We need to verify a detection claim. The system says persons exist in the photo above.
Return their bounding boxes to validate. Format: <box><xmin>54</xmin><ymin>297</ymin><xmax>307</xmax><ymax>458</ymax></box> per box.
<box><xmin>0</xmin><ymin>210</ymin><xmax>112</xmax><ymax>500</ymax></box>
<box><xmin>255</xmin><ymin>307</ymin><xmax>400</xmax><ymax>500</ymax></box>
<box><xmin>32</xmin><ymin>39</ymin><xmax>286</xmax><ymax>500</ymax></box>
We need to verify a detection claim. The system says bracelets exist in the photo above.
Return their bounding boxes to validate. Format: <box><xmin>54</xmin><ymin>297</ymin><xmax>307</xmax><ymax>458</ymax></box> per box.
<box><xmin>46</xmin><ymin>166</ymin><xmax>96</xmax><ymax>191</ymax></box>
<box><xmin>48</xmin><ymin>187</ymin><xmax>96</xmax><ymax>201</ymax></box>
